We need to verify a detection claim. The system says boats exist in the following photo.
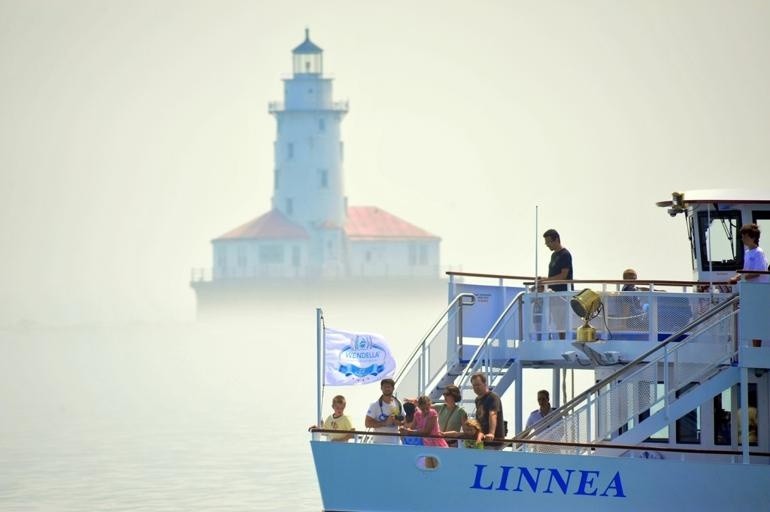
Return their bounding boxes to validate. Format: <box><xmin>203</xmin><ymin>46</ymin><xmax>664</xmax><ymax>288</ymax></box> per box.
<box><xmin>305</xmin><ymin>194</ymin><xmax>769</xmax><ymax>512</ymax></box>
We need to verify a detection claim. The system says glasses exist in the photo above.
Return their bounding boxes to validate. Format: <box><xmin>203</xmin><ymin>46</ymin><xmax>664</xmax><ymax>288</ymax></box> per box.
<box><xmin>443</xmin><ymin>392</ymin><xmax>452</xmax><ymax>396</ymax></box>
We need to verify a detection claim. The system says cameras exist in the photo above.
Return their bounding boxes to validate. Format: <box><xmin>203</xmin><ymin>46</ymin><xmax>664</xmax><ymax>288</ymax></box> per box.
<box><xmin>395</xmin><ymin>415</ymin><xmax>404</xmax><ymax>421</ymax></box>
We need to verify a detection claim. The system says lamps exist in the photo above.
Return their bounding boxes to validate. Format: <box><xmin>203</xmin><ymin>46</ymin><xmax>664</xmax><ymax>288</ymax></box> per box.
<box><xmin>570</xmin><ymin>287</ymin><xmax>605</xmax><ymax>343</ymax></box>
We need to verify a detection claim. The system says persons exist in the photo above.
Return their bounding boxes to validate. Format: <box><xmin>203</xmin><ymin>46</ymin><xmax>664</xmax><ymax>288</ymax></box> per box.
<box><xmin>733</xmin><ymin>405</ymin><xmax>758</xmax><ymax>447</ymax></box>
<box><xmin>529</xmin><ymin>285</ymin><xmax>551</xmax><ymax>340</ymax></box>
<box><xmin>539</xmin><ymin>229</ymin><xmax>576</xmax><ymax>339</ymax></box>
<box><xmin>365</xmin><ymin>374</ymin><xmax>507</xmax><ymax>450</ymax></box>
<box><xmin>618</xmin><ymin>269</ymin><xmax>657</xmax><ymax>329</ymax></box>
<box><xmin>522</xmin><ymin>390</ymin><xmax>566</xmax><ymax>454</ymax></box>
<box><xmin>309</xmin><ymin>396</ymin><xmax>355</xmax><ymax>441</ymax></box>
<box><xmin>731</xmin><ymin>225</ymin><xmax>767</xmax><ymax>283</ymax></box>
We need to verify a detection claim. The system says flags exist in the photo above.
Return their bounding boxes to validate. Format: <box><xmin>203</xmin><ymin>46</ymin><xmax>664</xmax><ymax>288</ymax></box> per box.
<box><xmin>319</xmin><ymin>325</ymin><xmax>399</xmax><ymax>392</ymax></box>
